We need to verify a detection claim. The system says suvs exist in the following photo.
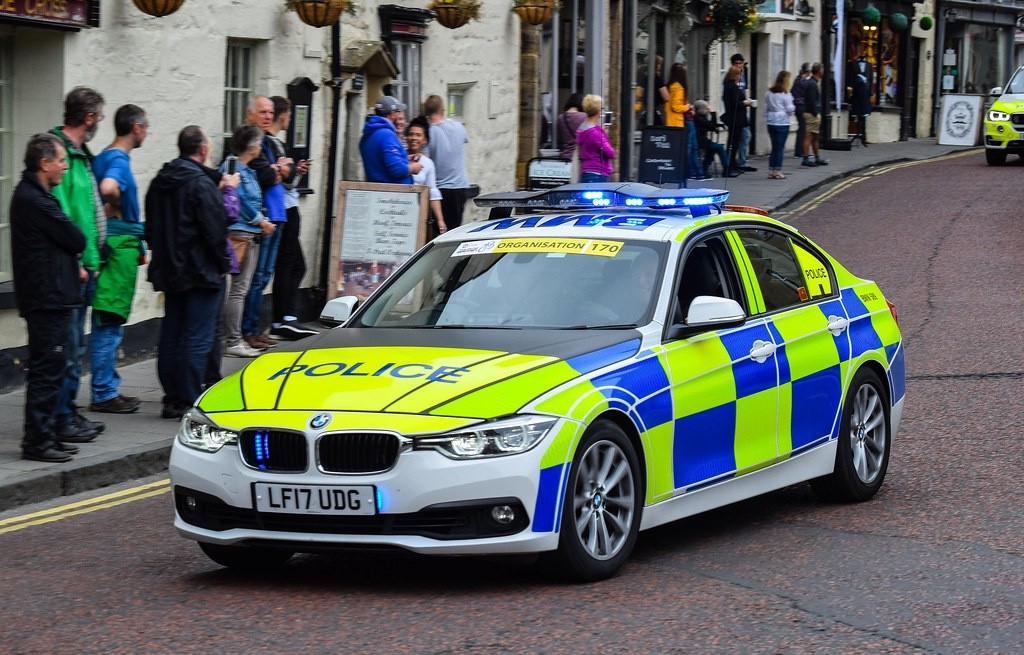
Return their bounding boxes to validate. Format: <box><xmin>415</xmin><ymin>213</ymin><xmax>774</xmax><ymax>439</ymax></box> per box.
<box><xmin>983</xmin><ymin>65</ymin><xmax>1024</xmax><ymax>166</ymax></box>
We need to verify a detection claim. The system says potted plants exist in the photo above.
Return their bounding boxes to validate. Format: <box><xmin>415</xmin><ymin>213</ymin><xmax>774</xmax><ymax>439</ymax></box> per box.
<box><xmin>285</xmin><ymin>0</ymin><xmax>357</xmax><ymax>28</ymax></box>
<box><xmin>511</xmin><ymin>0</ymin><xmax>565</xmax><ymax>25</ymax></box>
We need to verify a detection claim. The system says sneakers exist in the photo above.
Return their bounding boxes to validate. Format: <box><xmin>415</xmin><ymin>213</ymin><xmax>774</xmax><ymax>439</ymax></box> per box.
<box><xmin>118</xmin><ymin>395</ymin><xmax>139</xmax><ymax>403</ymax></box>
<box><xmin>255</xmin><ymin>333</ymin><xmax>279</xmax><ymax>347</ymax></box>
<box><xmin>73</xmin><ymin>414</ymin><xmax>104</xmax><ymax>432</ymax></box>
<box><xmin>245</xmin><ymin>337</ymin><xmax>269</xmax><ymax>351</ymax></box>
<box><xmin>90</xmin><ymin>397</ymin><xmax>139</xmax><ymax>413</ymax></box>
<box><xmin>55</xmin><ymin>424</ymin><xmax>97</xmax><ymax>442</ymax></box>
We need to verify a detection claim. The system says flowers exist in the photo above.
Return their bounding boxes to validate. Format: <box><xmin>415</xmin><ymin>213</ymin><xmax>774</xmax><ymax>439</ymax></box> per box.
<box><xmin>425</xmin><ymin>0</ymin><xmax>485</xmax><ymax>22</ymax></box>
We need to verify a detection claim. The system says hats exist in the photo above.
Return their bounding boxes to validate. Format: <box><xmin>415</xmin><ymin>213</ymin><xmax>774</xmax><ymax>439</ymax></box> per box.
<box><xmin>375</xmin><ymin>96</ymin><xmax>407</xmax><ymax>114</ymax></box>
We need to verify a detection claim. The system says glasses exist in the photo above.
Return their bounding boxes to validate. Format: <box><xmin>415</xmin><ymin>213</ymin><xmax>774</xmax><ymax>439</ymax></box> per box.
<box><xmin>94</xmin><ymin>114</ymin><xmax>106</xmax><ymax>121</ymax></box>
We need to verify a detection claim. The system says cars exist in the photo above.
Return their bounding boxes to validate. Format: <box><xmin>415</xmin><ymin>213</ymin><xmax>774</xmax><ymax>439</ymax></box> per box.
<box><xmin>166</xmin><ymin>180</ymin><xmax>908</xmax><ymax>584</ymax></box>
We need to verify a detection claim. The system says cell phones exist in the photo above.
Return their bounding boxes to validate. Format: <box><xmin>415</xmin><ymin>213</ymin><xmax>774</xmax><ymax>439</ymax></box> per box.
<box><xmin>605</xmin><ymin>112</ymin><xmax>613</xmax><ymax>126</ymax></box>
<box><xmin>227</xmin><ymin>157</ymin><xmax>237</xmax><ymax>176</ymax></box>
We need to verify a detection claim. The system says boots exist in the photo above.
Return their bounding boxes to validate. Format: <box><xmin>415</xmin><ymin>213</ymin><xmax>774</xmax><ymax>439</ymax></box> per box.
<box><xmin>723</xmin><ymin>167</ymin><xmax>738</xmax><ymax>177</ymax></box>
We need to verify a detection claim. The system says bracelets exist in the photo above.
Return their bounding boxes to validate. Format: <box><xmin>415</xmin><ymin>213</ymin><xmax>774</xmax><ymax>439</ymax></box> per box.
<box><xmin>275</xmin><ymin>162</ymin><xmax>281</xmax><ymax>170</ymax></box>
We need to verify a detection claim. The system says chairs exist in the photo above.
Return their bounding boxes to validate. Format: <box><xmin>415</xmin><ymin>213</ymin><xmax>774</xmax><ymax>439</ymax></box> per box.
<box><xmin>677</xmin><ymin>246</ymin><xmax>724</xmax><ymax>316</ymax></box>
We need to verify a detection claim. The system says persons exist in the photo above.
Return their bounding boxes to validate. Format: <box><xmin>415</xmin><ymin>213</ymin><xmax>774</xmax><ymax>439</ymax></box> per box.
<box><xmin>557</xmin><ymin>92</ymin><xmax>587</xmax><ymax>161</ymax></box>
<box><xmin>393</xmin><ymin>108</ymin><xmax>407</xmax><ymax>153</ymax></box>
<box><xmin>91</xmin><ymin>105</ymin><xmax>147</xmax><ymax>414</ymax></box>
<box><xmin>529</xmin><ymin>252</ymin><xmax>597</xmax><ymax>303</ymax></box>
<box><xmin>847</xmin><ymin>55</ymin><xmax>873</xmax><ymax>116</ymax></box>
<box><xmin>744</xmin><ymin>245</ymin><xmax>801</xmax><ymax>311</ymax></box>
<box><xmin>722</xmin><ymin>54</ymin><xmax>759</xmax><ymax>174</ymax></box>
<box><xmin>247</xmin><ymin>95</ymin><xmax>321</xmax><ymax>340</ymax></box>
<box><xmin>654</xmin><ymin>73</ymin><xmax>671</xmax><ymax>127</ymax></box>
<box><xmin>44</xmin><ymin>85</ymin><xmax>106</xmax><ymax>443</ymax></box>
<box><xmin>790</xmin><ymin>61</ymin><xmax>829</xmax><ymax>168</ymax></box>
<box><xmin>422</xmin><ymin>94</ymin><xmax>470</xmax><ymax>241</ymax></box>
<box><xmin>405</xmin><ymin>115</ymin><xmax>447</xmax><ymax>234</ymax></box>
<box><xmin>610</xmin><ymin>249</ymin><xmax>683</xmax><ymax>324</ymax></box>
<box><xmin>466</xmin><ymin>254</ymin><xmax>565</xmax><ymax>326</ymax></box>
<box><xmin>202</xmin><ymin>127</ymin><xmax>242</xmax><ymax>389</ymax></box>
<box><xmin>217</xmin><ymin>124</ymin><xmax>276</xmax><ymax>359</ymax></box>
<box><xmin>693</xmin><ymin>100</ymin><xmax>740</xmax><ymax>178</ymax></box>
<box><xmin>764</xmin><ymin>70</ymin><xmax>796</xmax><ymax>179</ymax></box>
<box><xmin>9</xmin><ymin>132</ymin><xmax>86</xmax><ymax>463</ymax></box>
<box><xmin>666</xmin><ymin>66</ymin><xmax>695</xmax><ymax>181</ymax></box>
<box><xmin>244</xmin><ymin>95</ymin><xmax>297</xmax><ymax>351</ymax></box>
<box><xmin>670</xmin><ymin>63</ymin><xmax>714</xmax><ymax>182</ymax></box>
<box><xmin>574</xmin><ymin>95</ymin><xmax>617</xmax><ymax>182</ymax></box>
<box><xmin>359</xmin><ymin>96</ymin><xmax>424</xmax><ymax>184</ymax></box>
<box><xmin>144</xmin><ymin>125</ymin><xmax>233</xmax><ymax>421</ymax></box>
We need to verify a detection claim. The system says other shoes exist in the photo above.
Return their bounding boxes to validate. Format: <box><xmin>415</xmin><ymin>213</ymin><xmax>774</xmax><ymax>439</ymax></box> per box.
<box><xmin>51</xmin><ymin>443</ymin><xmax>80</xmax><ymax>452</ymax></box>
<box><xmin>740</xmin><ymin>166</ymin><xmax>757</xmax><ymax>171</ymax></box>
<box><xmin>162</xmin><ymin>402</ymin><xmax>191</xmax><ymax>418</ymax></box>
<box><xmin>222</xmin><ymin>341</ymin><xmax>261</xmax><ymax>357</ymax></box>
<box><xmin>815</xmin><ymin>160</ymin><xmax>827</xmax><ymax>165</ymax></box>
<box><xmin>23</xmin><ymin>446</ymin><xmax>72</xmax><ymax>461</ymax></box>
<box><xmin>802</xmin><ymin>161</ymin><xmax>817</xmax><ymax>167</ymax></box>
<box><xmin>768</xmin><ymin>174</ymin><xmax>786</xmax><ymax>179</ymax></box>
<box><xmin>686</xmin><ymin>175</ymin><xmax>713</xmax><ymax>181</ymax></box>
<box><xmin>278</xmin><ymin>320</ymin><xmax>320</xmax><ymax>339</ymax></box>
